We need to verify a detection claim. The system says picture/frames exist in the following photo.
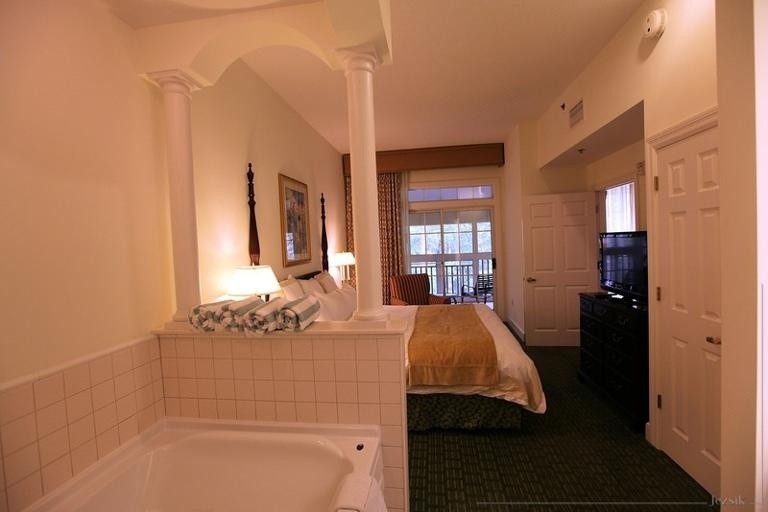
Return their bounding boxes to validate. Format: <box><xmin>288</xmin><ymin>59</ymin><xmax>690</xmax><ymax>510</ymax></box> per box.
<box><xmin>278</xmin><ymin>172</ymin><xmax>311</xmax><ymax>268</ymax></box>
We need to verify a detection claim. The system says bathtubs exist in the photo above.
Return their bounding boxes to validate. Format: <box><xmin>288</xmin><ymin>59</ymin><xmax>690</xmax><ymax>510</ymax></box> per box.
<box><xmin>22</xmin><ymin>414</ymin><xmax>386</xmax><ymax>512</ymax></box>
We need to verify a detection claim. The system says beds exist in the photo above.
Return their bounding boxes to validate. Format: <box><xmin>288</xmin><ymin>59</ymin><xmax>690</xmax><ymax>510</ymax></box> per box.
<box><xmin>295</xmin><ymin>270</ymin><xmax>537</xmax><ymax>436</ymax></box>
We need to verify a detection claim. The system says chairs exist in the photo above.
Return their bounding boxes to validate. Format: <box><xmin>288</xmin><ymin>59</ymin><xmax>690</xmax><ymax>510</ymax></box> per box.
<box><xmin>461</xmin><ymin>274</ymin><xmax>494</xmax><ymax>303</ymax></box>
<box><xmin>389</xmin><ymin>274</ymin><xmax>451</xmax><ymax>306</ymax></box>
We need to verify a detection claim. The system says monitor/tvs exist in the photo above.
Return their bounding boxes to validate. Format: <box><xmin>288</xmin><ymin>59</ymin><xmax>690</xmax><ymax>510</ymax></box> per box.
<box><xmin>598</xmin><ymin>230</ymin><xmax>648</xmax><ymax>306</ymax></box>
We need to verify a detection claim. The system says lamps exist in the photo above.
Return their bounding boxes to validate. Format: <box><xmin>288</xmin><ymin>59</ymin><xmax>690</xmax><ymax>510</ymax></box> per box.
<box><xmin>228</xmin><ymin>265</ymin><xmax>281</xmax><ymax>297</ymax></box>
<box><xmin>333</xmin><ymin>252</ymin><xmax>355</xmax><ymax>283</ymax></box>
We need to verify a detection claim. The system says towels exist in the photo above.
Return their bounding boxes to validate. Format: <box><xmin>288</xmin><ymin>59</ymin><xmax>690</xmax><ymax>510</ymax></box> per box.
<box><xmin>214</xmin><ymin>295</ymin><xmax>264</xmax><ymax>332</ymax></box>
<box><xmin>244</xmin><ymin>297</ymin><xmax>286</xmax><ymax>335</ymax></box>
<box><xmin>188</xmin><ymin>300</ymin><xmax>234</xmax><ymax>334</ymax></box>
<box><xmin>280</xmin><ymin>293</ymin><xmax>320</xmax><ymax>332</ymax></box>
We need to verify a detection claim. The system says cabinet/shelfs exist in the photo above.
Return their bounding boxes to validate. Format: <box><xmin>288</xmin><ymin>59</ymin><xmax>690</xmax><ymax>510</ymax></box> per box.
<box><xmin>578</xmin><ymin>292</ymin><xmax>649</xmax><ymax>432</ymax></box>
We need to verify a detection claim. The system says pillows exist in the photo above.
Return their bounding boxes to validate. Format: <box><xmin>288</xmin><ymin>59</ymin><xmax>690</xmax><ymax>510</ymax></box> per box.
<box><xmin>279</xmin><ymin>274</ymin><xmax>357</xmax><ymax>322</ymax></box>
